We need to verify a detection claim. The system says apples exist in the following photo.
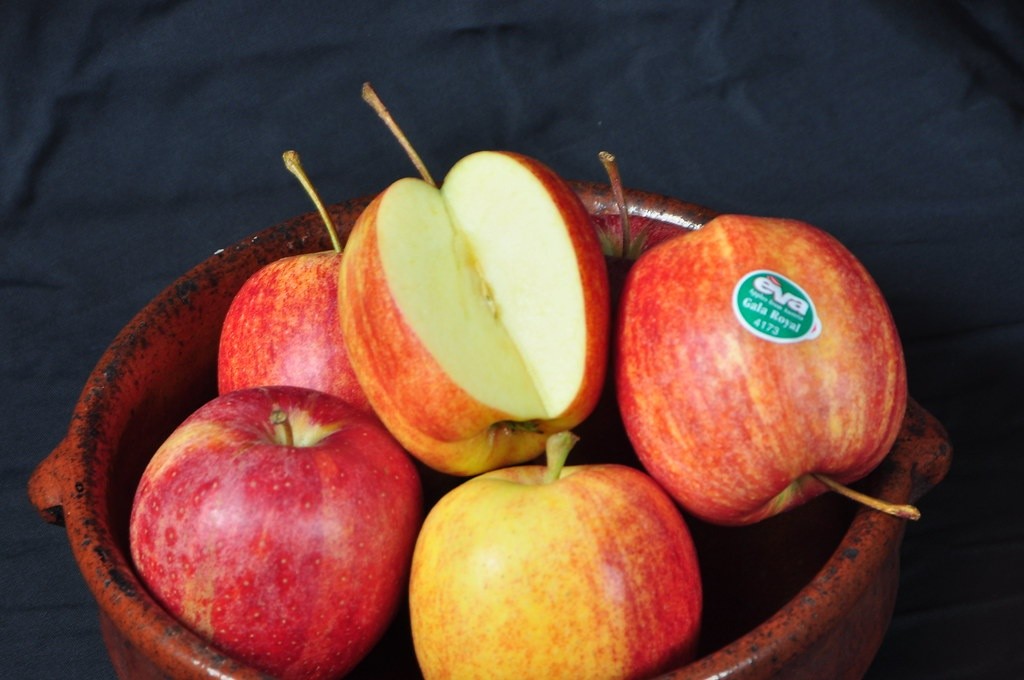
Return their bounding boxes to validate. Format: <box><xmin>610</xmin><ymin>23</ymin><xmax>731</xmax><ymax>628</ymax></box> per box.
<box><xmin>129</xmin><ymin>82</ymin><xmax>919</xmax><ymax>680</ymax></box>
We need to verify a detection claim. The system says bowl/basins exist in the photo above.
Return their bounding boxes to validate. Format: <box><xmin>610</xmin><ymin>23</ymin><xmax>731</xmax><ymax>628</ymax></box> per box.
<box><xmin>28</xmin><ymin>186</ymin><xmax>949</xmax><ymax>679</ymax></box>
